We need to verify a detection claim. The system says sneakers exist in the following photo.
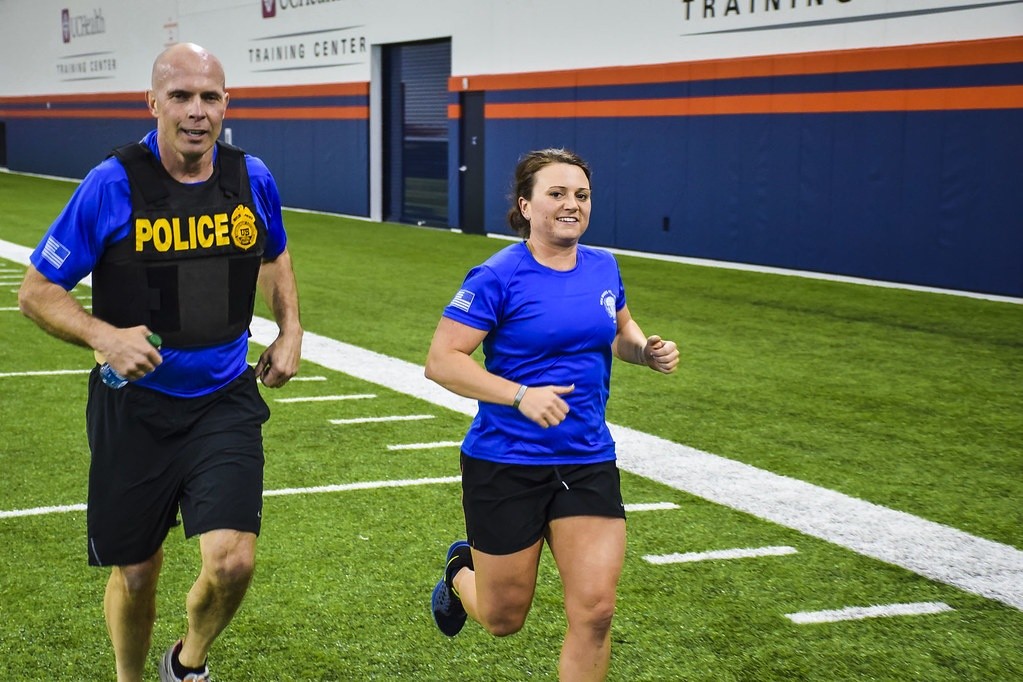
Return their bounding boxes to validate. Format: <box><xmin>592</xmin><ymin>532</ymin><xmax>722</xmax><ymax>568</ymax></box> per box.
<box><xmin>432</xmin><ymin>541</ymin><xmax>473</xmax><ymax>637</ymax></box>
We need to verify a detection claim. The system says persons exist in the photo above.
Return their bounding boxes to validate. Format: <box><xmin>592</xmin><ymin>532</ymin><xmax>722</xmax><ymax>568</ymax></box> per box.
<box><xmin>20</xmin><ymin>44</ymin><xmax>307</xmax><ymax>682</ymax></box>
<box><xmin>423</xmin><ymin>145</ymin><xmax>680</xmax><ymax>682</ymax></box>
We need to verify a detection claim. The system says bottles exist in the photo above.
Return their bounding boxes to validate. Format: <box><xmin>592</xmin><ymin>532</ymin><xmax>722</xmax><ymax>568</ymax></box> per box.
<box><xmin>99</xmin><ymin>334</ymin><xmax>161</xmax><ymax>388</ymax></box>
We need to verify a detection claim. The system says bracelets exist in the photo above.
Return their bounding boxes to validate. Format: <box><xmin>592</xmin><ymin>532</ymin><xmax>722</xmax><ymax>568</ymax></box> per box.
<box><xmin>512</xmin><ymin>385</ymin><xmax>527</xmax><ymax>408</ymax></box>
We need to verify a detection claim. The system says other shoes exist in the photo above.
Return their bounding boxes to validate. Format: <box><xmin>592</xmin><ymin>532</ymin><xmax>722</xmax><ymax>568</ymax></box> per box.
<box><xmin>158</xmin><ymin>640</ymin><xmax>211</xmax><ymax>682</ymax></box>
